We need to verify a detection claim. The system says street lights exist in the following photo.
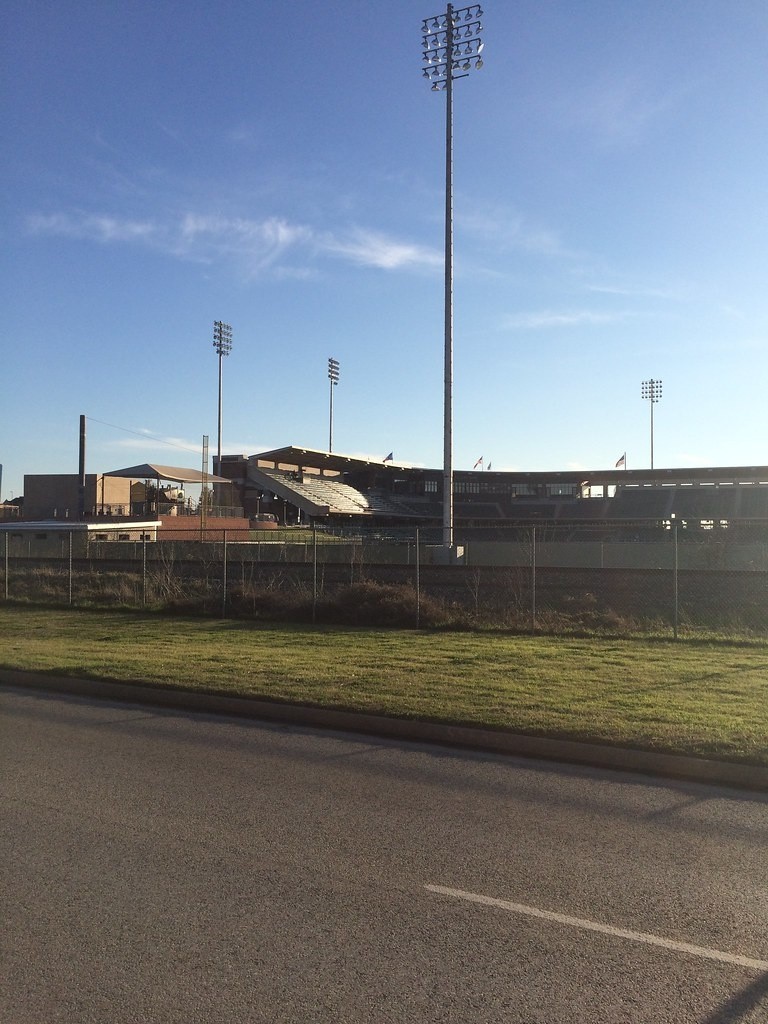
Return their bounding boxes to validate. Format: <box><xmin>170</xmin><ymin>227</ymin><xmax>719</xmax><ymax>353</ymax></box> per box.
<box><xmin>419</xmin><ymin>1</ymin><xmax>482</xmax><ymax>544</ymax></box>
<box><xmin>212</xmin><ymin>321</ymin><xmax>233</xmax><ymax>506</ymax></box>
<box><xmin>328</xmin><ymin>357</ymin><xmax>339</xmax><ymax>454</ymax></box>
<box><xmin>641</xmin><ymin>378</ymin><xmax>661</xmax><ymax>468</ymax></box>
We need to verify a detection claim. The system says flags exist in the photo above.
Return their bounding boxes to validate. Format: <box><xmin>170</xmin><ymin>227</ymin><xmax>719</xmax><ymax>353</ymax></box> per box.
<box><xmin>385</xmin><ymin>453</ymin><xmax>393</xmax><ymax>460</ymax></box>
<box><xmin>616</xmin><ymin>456</ymin><xmax>625</xmax><ymax>468</ymax></box>
<box><xmin>474</xmin><ymin>457</ymin><xmax>482</xmax><ymax>469</ymax></box>
